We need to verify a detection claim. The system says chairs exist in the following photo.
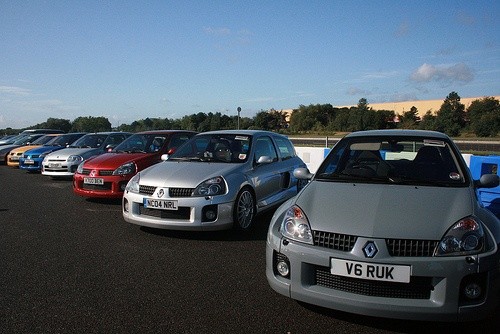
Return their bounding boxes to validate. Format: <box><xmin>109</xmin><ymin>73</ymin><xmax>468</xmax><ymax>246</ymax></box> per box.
<box><xmin>229</xmin><ymin>141</ymin><xmax>243</xmax><ymax>159</ymax></box>
<box><xmin>409</xmin><ymin>146</ymin><xmax>445</xmax><ymax>184</ymax></box>
<box><xmin>213</xmin><ymin>140</ymin><xmax>231</xmax><ymax>163</ymax></box>
<box><xmin>347</xmin><ymin>150</ymin><xmax>383</xmax><ymax>176</ymax></box>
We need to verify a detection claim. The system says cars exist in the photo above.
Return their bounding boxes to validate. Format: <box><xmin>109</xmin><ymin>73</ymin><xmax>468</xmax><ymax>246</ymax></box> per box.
<box><xmin>265</xmin><ymin>129</ymin><xmax>500</xmax><ymax>323</ymax></box>
<box><xmin>19</xmin><ymin>133</ymin><xmax>87</xmax><ymax>172</ymax></box>
<box><xmin>41</xmin><ymin>132</ymin><xmax>134</xmax><ymax>181</ymax></box>
<box><xmin>0</xmin><ymin>128</ymin><xmax>66</xmax><ymax>168</ymax></box>
<box><xmin>121</xmin><ymin>129</ymin><xmax>310</xmax><ymax>235</ymax></box>
<box><xmin>72</xmin><ymin>131</ymin><xmax>199</xmax><ymax>200</ymax></box>
